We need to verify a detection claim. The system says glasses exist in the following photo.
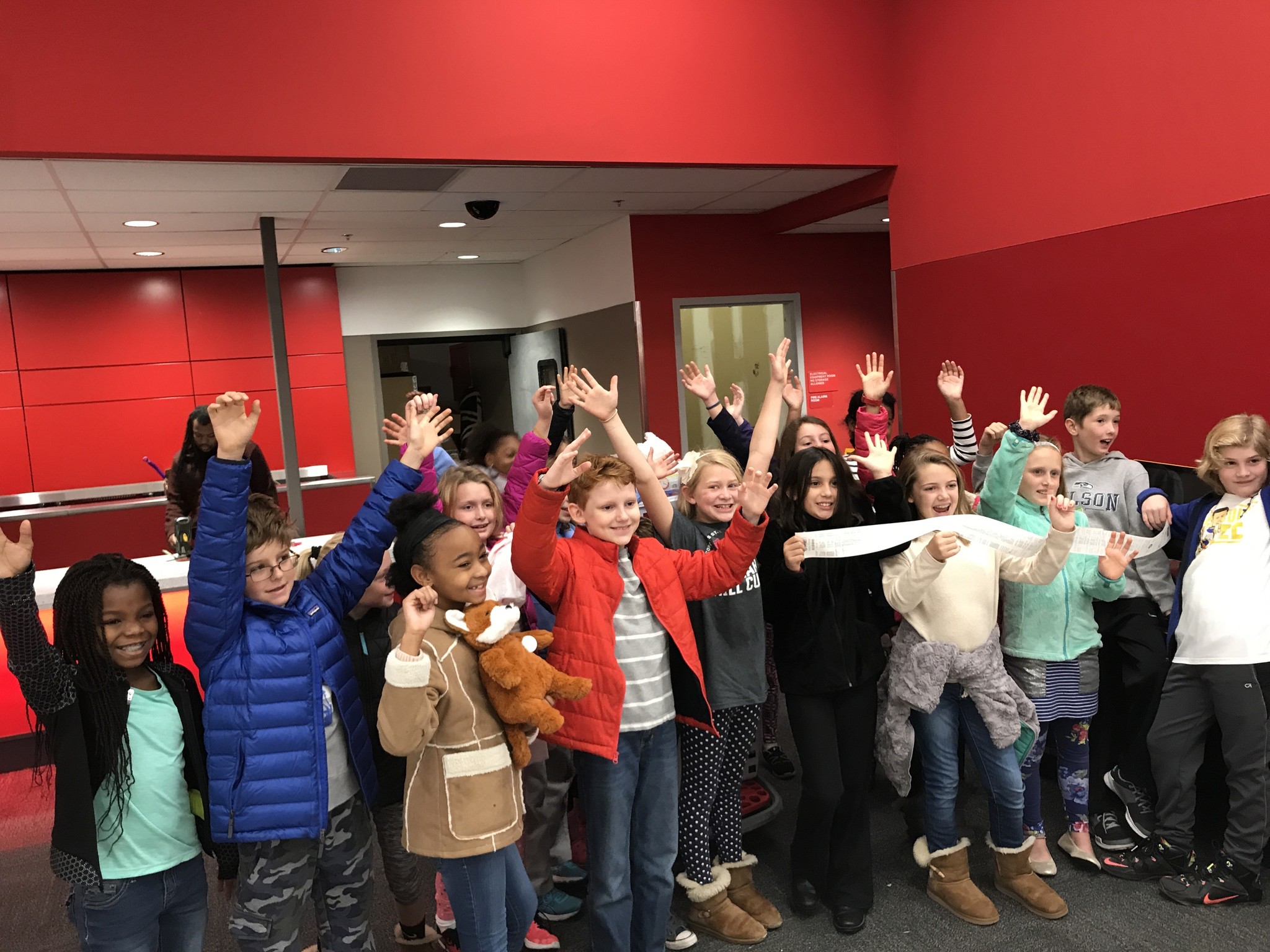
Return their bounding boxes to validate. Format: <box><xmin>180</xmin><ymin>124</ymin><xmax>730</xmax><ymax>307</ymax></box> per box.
<box><xmin>245</xmin><ymin>549</ymin><xmax>300</xmax><ymax>582</ymax></box>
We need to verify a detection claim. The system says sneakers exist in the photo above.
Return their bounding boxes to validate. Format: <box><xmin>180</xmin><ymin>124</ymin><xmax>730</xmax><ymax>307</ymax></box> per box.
<box><xmin>524</xmin><ymin>910</ymin><xmax>560</xmax><ymax>950</ymax></box>
<box><xmin>665</xmin><ymin>910</ymin><xmax>698</xmax><ymax>950</ymax></box>
<box><xmin>536</xmin><ymin>886</ymin><xmax>583</xmax><ymax>922</ymax></box>
<box><xmin>551</xmin><ymin>860</ymin><xmax>588</xmax><ymax>883</ymax></box>
<box><xmin>436</xmin><ymin>926</ymin><xmax>460</xmax><ymax>952</ymax></box>
<box><xmin>1159</xmin><ymin>854</ymin><xmax>1262</xmax><ymax>906</ymax></box>
<box><xmin>1103</xmin><ymin>764</ymin><xmax>1159</xmax><ymax>839</ymax></box>
<box><xmin>1099</xmin><ymin>836</ymin><xmax>1199</xmax><ymax>881</ymax></box>
<box><xmin>1089</xmin><ymin>811</ymin><xmax>1135</xmax><ymax>850</ymax></box>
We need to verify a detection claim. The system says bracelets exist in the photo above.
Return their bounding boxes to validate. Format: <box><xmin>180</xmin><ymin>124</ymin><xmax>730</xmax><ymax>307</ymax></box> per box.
<box><xmin>706</xmin><ymin>399</ymin><xmax>721</xmax><ymax>410</ymax></box>
<box><xmin>862</xmin><ymin>392</ymin><xmax>883</xmax><ymax>408</ymax></box>
<box><xmin>599</xmin><ymin>409</ymin><xmax>617</xmax><ymax>423</ymax></box>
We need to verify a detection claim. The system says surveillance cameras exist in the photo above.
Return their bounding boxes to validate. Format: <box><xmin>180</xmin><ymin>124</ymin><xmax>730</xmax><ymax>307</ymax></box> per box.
<box><xmin>465</xmin><ymin>199</ymin><xmax>500</xmax><ymax>220</ymax></box>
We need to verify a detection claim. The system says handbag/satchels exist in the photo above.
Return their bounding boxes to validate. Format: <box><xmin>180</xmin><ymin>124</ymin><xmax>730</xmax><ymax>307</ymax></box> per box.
<box><xmin>612</xmin><ymin>432</ymin><xmax>679</xmax><ymax>507</ymax></box>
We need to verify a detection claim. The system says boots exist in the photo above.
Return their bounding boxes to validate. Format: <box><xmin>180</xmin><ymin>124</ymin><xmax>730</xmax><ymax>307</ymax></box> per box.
<box><xmin>912</xmin><ymin>835</ymin><xmax>1000</xmax><ymax>925</ymax></box>
<box><xmin>676</xmin><ymin>866</ymin><xmax>767</xmax><ymax>944</ymax></box>
<box><xmin>713</xmin><ymin>850</ymin><xmax>783</xmax><ymax>929</ymax></box>
<box><xmin>985</xmin><ymin>831</ymin><xmax>1068</xmax><ymax>919</ymax></box>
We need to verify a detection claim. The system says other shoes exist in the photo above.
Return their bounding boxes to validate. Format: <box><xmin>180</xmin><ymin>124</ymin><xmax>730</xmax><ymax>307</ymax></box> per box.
<box><xmin>761</xmin><ymin>745</ymin><xmax>796</xmax><ymax>779</ymax></box>
<box><xmin>1057</xmin><ymin>831</ymin><xmax>1101</xmax><ymax>872</ymax></box>
<box><xmin>834</xmin><ymin>906</ymin><xmax>865</xmax><ymax>932</ymax></box>
<box><xmin>393</xmin><ymin>922</ymin><xmax>448</xmax><ymax>952</ymax></box>
<box><xmin>792</xmin><ymin>876</ymin><xmax>818</xmax><ymax>914</ymax></box>
<box><xmin>1028</xmin><ymin>837</ymin><xmax>1057</xmax><ymax>876</ymax></box>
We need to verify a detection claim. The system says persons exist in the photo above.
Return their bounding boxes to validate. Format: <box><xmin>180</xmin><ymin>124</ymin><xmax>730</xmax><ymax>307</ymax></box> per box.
<box><xmin>1</xmin><ymin>337</ymin><xmax>1169</xmax><ymax>952</ymax></box>
<box><xmin>1095</xmin><ymin>414</ymin><xmax>1270</xmax><ymax>907</ymax></box>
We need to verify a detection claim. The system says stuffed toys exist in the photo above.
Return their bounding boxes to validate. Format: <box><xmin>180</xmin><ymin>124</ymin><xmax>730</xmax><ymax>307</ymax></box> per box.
<box><xmin>443</xmin><ymin>601</ymin><xmax>590</xmax><ymax>770</ymax></box>
